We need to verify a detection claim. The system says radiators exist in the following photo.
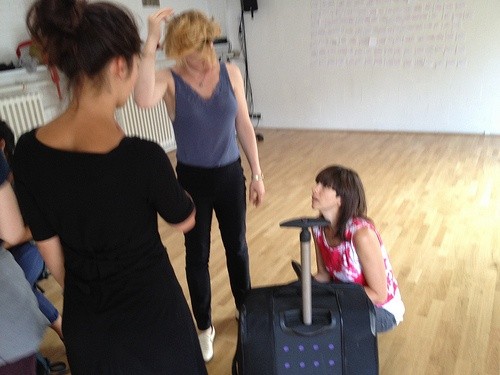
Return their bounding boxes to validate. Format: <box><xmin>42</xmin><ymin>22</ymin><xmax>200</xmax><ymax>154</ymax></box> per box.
<box><xmin>0</xmin><ymin>51</ymin><xmax>177</xmax><ymax>154</ymax></box>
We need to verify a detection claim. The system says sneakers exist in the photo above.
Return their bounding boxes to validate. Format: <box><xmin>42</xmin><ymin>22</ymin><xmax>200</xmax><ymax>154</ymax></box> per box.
<box><xmin>196</xmin><ymin>323</ymin><xmax>216</xmax><ymax>362</ymax></box>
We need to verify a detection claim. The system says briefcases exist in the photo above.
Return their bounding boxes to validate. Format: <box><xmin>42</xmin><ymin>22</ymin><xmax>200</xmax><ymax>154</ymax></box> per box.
<box><xmin>231</xmin><ymin>219</ymin><xmax>379</xmax><ymax>375</ymax></box>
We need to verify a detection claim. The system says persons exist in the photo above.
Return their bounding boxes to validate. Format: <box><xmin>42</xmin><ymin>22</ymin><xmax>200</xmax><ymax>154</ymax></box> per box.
<box><xmin>0</xmin><ymin>119</ymin><xmax>64</xmax><ymax>375</ymax></box>
<box><xmin>11</xmin><ymin>0</ymin><xmax>207</xmax><ymax>375</ymax></box>
<box><xmin>134</xmin><ymin>8</ymin><xmax>265</xmax><ymax>362</ymax></box>
<box><xmin>312</xmin><ymin>165</ymin><xmax>405</xmax><ymax>334</ymax></box>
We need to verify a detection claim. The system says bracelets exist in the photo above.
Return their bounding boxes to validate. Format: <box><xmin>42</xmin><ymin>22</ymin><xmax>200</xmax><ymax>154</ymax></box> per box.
<box><xmin>251</xmin><ymin>174</ymin><xmax>265</xmax><ymax>181</ymax></box>
